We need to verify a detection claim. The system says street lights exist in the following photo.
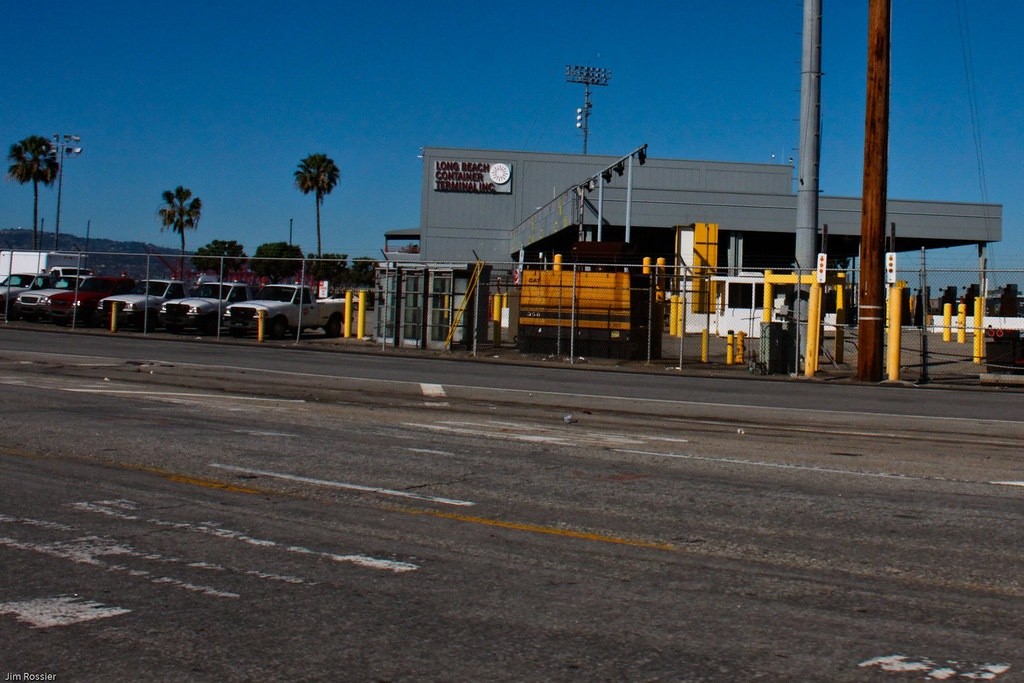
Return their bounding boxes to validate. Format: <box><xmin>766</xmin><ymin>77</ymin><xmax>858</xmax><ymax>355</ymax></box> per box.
<box><xmin>565</xmin><ymin>65</ymin><xmax>611</xmax><ymax>155</ymax></box>
<box><xmin>42</xmin><ymin>134</ymin><xmax>83</xmax><ymax>251</ymax></box>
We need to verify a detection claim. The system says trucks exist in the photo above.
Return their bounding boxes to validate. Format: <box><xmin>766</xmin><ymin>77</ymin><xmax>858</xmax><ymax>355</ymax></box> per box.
<box><xmin>0</xmin><ymin>252</ymin><xmax>93</xmax><ymax>283</ymax></box>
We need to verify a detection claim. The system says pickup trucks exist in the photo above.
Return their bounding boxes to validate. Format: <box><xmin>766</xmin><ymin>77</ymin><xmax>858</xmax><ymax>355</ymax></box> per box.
<box><xmin>0</xmin><ymin>266</ymin><xmax>136</xmax><ymax>328</ymax></box>
<box><xmin>157</xmin><ymin>280</ymin><xmax>255</xmax><ymax>334</ymax></box>
<box><xmin>223</xmin><ymin>283</ymin><xmax>346</xmax><ymax>340</ymax></box>
<box><xmin>96</xmin><ymin>278</ymin><xmax>192</xmax><ymax>333</ymax></box>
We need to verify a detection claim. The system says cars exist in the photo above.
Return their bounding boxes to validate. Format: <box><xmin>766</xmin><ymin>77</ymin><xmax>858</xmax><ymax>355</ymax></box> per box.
<box><xmin>316</xmin><ymin>292</ymin><xmax>368</xmax><ymax>311</ymax></box>
